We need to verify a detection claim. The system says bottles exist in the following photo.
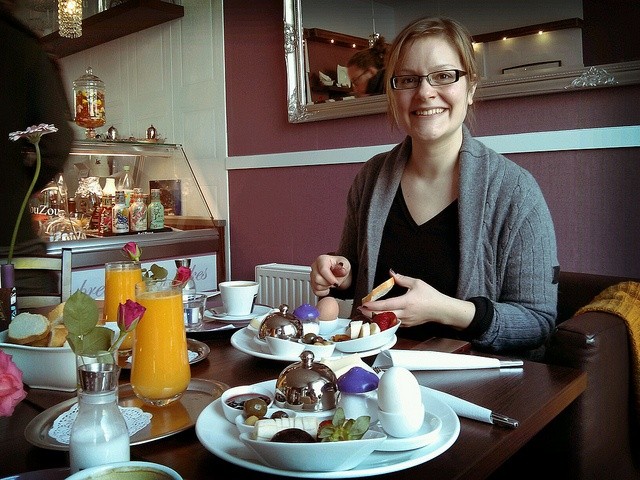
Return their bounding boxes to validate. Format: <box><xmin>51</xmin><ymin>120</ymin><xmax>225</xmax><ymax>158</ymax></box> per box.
<box><xmin>118</xmin><ymin>166</ymin><xmax>134</xmax><ymax>204</ymax></box>
<box><xmin>98</xmin><ymin>194</ymin><xmax>117</xmax><ymax>236</ymax></box>
<box><xmin>130</xmin><ymin>188</ymin><xmax>147</xmax><ymax>231</ymax></box>
<box><xmin>103</xmin><ymin>177</ymin><xmax>118</xmax><ymax>209</ymax></box>
<box><xmin>71</xmin><ymin>363</ymin><xmax>132</xmax><ymax>475</ymax></box>
<box><xmin>148</xmin><ymin>188</ymin><xmax>164</xmax><ymax>229</ymax></box>
<box><xmin>173</xmin><ymin>258</ymin><xmax>195</xmax><ymax>326</ymax></box>
<box><xmin>112</xmin><ymin>190</ymin><xmax>129</xmax><ymax>233</ymax></box>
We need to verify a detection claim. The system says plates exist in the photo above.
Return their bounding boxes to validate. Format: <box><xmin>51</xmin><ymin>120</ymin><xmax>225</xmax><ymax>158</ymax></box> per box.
<box><xmin>195</xmin><ymin>378</ymin><xmax>460</xmax><ymax>479</ymax></box>
<box><xmin>205</xmin><ymin>304</ymin><xmax>274</xmax><ymax>324</ymax></box>
<box><xmin>24</xmin><ymin>378</ymin><xmax>231</xmax><ymax>452</ymax></box>
<box><xmin>328</xmin><ymin>319</ymin><xmax>401</xmax><ymax>353</ymax></box>
<box><xmin>239</xmin><ymin>416</ymin><xmax>387</xmax><ymax>472</ymax></box>
<box><xmin>230</xmin><ymin>319</ymin><xmax>398</xmax><ymax>361</ymax></box>
<box><xmin>118</xmin><ymin>337</ymin><xmax>210</xmax><ymax>369</ymax></box>
<box><xmin>371</xmin><ymin>412</ymin><xmax>442</xmax><ymax>453</ymax></box>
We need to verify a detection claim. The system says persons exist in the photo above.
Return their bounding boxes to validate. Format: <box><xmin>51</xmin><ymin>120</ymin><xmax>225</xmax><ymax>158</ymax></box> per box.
<box><xmin>310</xmin><ymin>16</ymin><xmax>560</xmax><ymax>354</ymax></box>
<box><xmin>348</xmin><ymin>37</ymin><xmax>385</xmax><ymax>98</ymax></box>
<box><xmin>1</xmin><ymin>7</ymin><xmax>74</xmax><ymax>296</ymax></box>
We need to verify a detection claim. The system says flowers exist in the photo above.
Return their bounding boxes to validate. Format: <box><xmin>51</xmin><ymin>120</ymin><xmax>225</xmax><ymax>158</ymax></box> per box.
<box><xmin>120</xmin><ymin>241</ymin><xmax>142</xmax><ymax>262</ymax></box>
<box><xmin>109</xmin><ymin>299</ymin><xmax>146</xmax><ymax>351</ymax></box>
<box><xmin>0</xmin><ymin>123</ymin><xmax>58</xmax><ymax>259</ymax></box>
<box><xmin>171</xmin><ymin>266</ymin><xmax>192</xmax><ymax>289</ymax></box>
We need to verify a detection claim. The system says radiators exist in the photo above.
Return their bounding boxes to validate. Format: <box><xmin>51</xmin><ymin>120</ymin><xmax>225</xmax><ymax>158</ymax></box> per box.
<box><xmin>255</xmin><ymin>262</ymin><xmax>320</xmax><ymax>311</ymax></box>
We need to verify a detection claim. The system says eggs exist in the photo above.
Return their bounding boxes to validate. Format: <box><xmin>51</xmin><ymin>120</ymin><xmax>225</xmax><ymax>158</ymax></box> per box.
<box><xmin>315</xmin><ymin>296</ymin><xmax>340</xmax><ymax>321</ymax></box>
<box><xmin>377</xmin><ymin>367</ymin><xmax>421</xmax><ymax>413</ymax></box>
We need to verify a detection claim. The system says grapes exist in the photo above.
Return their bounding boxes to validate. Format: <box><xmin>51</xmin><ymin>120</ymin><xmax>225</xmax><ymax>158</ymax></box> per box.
<box><xmin>244</xmin><ymin>398</ymin><xmax>266</xmax><ymax>418</ymax></box>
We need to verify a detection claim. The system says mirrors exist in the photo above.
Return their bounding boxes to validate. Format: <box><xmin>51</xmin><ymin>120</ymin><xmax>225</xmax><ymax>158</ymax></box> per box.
<box><xmin>281</xmin><ymin>0</ymin><xmax>640</xmax><ymax>124</ymax></box>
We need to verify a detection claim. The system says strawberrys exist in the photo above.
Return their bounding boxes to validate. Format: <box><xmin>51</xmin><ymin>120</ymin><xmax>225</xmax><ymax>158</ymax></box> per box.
<box><xmin>317</xmin><ymin>407</ymin><xmax>370</xmax><ymax>443</ymax></box>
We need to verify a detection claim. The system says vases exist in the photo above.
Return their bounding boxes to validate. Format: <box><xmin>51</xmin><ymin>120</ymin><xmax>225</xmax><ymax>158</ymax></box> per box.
<box><xmin>76</xmin><ymin>351</ymin><xmax>118</xmax><ymax>393</ymax></box>
<box><xmin>1</xmin><ymin>262</ymin><xmax>19</xmax><ymax>330</ymax></box>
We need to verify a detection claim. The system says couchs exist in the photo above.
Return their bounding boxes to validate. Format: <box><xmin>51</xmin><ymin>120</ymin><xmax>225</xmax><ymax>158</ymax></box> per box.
<box><xmin>555</xmin><ymin>266</ymin><xmax>636</xmax><ymax>476</ymax></box>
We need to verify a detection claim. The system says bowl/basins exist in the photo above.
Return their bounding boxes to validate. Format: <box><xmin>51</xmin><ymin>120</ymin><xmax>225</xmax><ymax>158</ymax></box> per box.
<box><xmin>235</xmin><ymin>409</ymin><xmax>297</xmax><ymax>433</ymax></box>
<box><xmin>221</xmin><ymin>385</ymin><xmax>274</xmax><ymax>425</ymax></box>
<box><xmin>378</xmin><ymin>406</ymin><xmax>424</xmax><ymax>437</ymax></box>
<box><xmin>247</xmin><ymin>323</ymin><xmax>261</xmax><ymax>336</ymax></box>
<box><xmin>267</xmin><ymin>337</ymin><xmax>305</xmax><ymax>358</ymax></box>
<box><xmin>65</xmin><ymin>461</ymin><xmax>184</xmax><ymax>480</ymax></box>
<box><xmin>304</xmin><ymin>341</ymin><xmax>336</xmax><ymax>357</ymax></box>
<box><xmin>255</xmin><ymin>337</ymin><xmax>270</xmax><ymax>353</ymax></box>
<box><xmin>1</xmin><ymin>319</ymin><xmax>122</xmax><ymax>392</ymax></box>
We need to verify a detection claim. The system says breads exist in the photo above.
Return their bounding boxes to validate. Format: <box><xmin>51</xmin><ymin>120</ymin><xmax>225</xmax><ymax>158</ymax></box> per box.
<box><xmin>10</xmin><ymin>302</ymin><xmax>75</xmax><ymax>353</ymax></box>
<box><xmin>362</xmin><ymin>276</ymin><xmax>396</xmax><ymax>303</ymax></box>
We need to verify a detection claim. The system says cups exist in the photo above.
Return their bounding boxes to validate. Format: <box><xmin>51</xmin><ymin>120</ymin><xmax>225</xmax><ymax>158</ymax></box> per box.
<box><xmin>130</xmin><ymin>279</ymin><xmax>191</xmax><ymax>407</ymax></box>
<box><xmin>183</xmin><ymin>294</ymin><xmax>206</xmax><ymax>331</ymax></box>
<box><xmin>105</xmin><ymin>261</ymin><xmax>143</xmax><ymax>359</ymax></box>
<box><xmin>218</xmin><ymin>280</ymin><xmax>259</xmax><ymax>316</ymax></box>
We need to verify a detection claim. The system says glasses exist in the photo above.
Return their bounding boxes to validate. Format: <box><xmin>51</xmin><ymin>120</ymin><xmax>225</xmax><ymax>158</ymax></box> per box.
<box><xmin>351</xmin><ymin>69</ymin><xmax>367</xmax><ymax>90</ymax></box>
<box><xmin>390</xmin><ymin>69</ymin><xmax>466</xmax><ymax>91</ymax></box>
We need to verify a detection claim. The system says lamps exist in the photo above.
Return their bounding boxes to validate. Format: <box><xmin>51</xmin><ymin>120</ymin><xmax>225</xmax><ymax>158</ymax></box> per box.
<box><xmin>59</xmin><ymin>0</ymin><xmax>83</xmax><ymax>39</ymax></box>
